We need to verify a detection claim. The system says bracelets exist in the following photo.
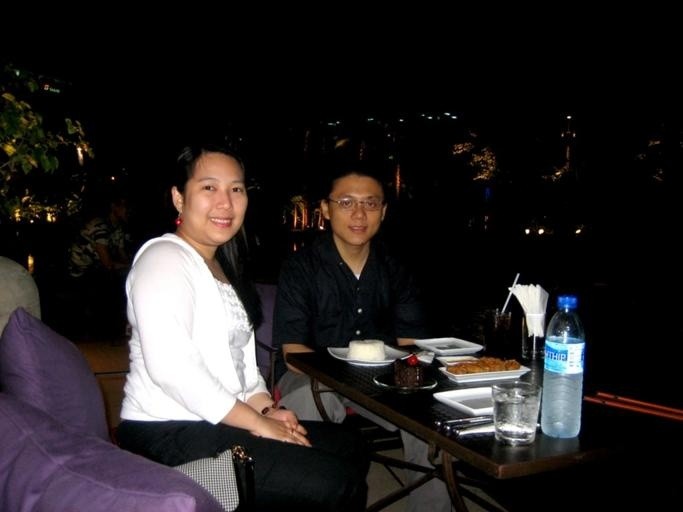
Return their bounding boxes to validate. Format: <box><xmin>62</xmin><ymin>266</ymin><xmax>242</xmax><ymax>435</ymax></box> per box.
<box><xmin>260</xmin><ymin>402</ymin><xmax>286</xmax><ymax>417</ymax></box>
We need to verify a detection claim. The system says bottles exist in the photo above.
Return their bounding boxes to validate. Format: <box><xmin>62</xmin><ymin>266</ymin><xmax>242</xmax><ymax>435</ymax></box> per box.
<box><xmin>540</xmin><ymin>297</ymin><xmax>586</xmax><ymax>439</ymax></box>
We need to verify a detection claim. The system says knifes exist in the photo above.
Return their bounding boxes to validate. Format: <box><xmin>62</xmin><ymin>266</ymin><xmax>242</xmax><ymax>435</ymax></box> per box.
<box><xmin>434</xmin><ymin>416</ymin><xmax>543</xmax><ymax>430</ymax></box>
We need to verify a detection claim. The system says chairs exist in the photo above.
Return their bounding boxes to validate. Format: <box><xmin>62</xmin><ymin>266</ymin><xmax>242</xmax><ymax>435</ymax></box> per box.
<box><xmin>246</xmin><ymin>275</ymin><xmax>404</xmax><ymax>490</ymax></box>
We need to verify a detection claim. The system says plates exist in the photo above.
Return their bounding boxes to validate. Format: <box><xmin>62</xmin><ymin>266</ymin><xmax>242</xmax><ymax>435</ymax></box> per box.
<box><xmin>432</xmin><ymin>384</ymin><xmax>495</xmax><ymax>416</ymax></box>
<box><xmin>325</xmin><ymin>332</ymin><xmax>531</xmax><ymax>394</ymax></box>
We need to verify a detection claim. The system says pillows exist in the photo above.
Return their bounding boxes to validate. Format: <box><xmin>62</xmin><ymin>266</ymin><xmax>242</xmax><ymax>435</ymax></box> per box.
<box><xmin>0</xmin><ymin>392</ymin><xmax>225</xmax><ymax>511</ymax></box>
<box><xmin>0</xmin><ymin>306</ymin><xmax>113</xmax><ymax>444</ymax></box>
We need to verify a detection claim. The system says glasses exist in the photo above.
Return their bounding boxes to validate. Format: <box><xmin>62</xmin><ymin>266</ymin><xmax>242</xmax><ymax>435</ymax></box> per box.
<box><xmin>329</xmin><ymin>198</ymin><xmax>382</xmax><ymax>211</ymax></box>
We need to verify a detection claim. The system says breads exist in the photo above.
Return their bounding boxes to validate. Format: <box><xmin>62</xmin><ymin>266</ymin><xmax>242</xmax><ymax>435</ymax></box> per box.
<box><xmin>447</xmin><ymin>357</ymin><xmax>519</xmax><ymax>375</ymax></box>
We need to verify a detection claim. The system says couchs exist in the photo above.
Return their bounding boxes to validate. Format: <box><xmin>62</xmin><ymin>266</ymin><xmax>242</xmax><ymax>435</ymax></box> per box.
<box><xmin>0</xmin><ymin>256</ymin><xmax>241</xmax><ymax>511</ymax></box>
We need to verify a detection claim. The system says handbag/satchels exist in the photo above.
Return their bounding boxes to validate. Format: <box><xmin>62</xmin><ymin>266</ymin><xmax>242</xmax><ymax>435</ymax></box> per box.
<box><xmin>172</xmin><ymin>444</ymin><xmax>256</xmax><ymax>511</ymax></box>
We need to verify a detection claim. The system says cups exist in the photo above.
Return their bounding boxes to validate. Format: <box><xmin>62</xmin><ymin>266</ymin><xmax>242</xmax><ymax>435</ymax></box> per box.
<box><xmin>492</xmin><ymin>379</ymin><xmax>542</xmax><ymax>447</ymax></box>
<box><xmin>488</xmin><ymin>309</ymin><xmax>511</xmax><ymax>330</ymax></box>
<box><xmin>520</xmin><ymin>315</ymin><xmax>544</xmax><ymax>358</ymax></box>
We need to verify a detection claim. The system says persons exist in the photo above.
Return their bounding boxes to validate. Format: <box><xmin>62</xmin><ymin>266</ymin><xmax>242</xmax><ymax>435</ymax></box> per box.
<box><xmin>115</xmin><ymin>146</ymin><xmax>368</xmax><ymax>512</ymax></box>
<box><xmin>61</xmin><ymin>198</ymin><xmax>131</xmax><ymax>284</ymax></box>
<box><xmin>271</xmin><ymin>169</ymin><xmax>455</xmax><ymax>512</ymax></box>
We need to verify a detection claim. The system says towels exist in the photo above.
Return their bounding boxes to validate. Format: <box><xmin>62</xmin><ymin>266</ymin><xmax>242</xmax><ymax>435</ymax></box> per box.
<box><xmin>507</xmin><ymin>283</ymin><xmax>549</xmax><ymax>339</ymax></box>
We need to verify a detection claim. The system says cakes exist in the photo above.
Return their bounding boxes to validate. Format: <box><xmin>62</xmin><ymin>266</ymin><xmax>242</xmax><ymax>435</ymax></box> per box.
<box><xmin>347</xmin><ymin>339</ymin><xmax>385</xmax><ymax>361</ymax></box>
<box><xmin>388</xmin><ymin>355</ymin><xmax>424</xmax><ymax>386</ymax></box>
